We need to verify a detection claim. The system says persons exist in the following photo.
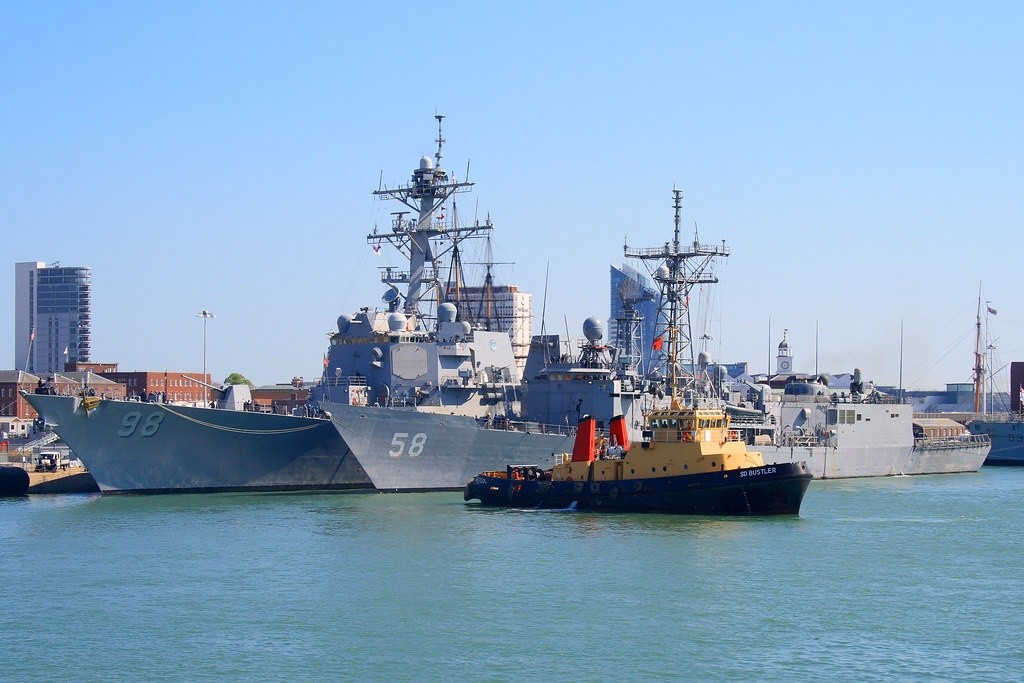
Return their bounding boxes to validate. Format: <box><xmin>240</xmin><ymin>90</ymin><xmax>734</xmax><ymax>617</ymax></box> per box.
<box><xmin>272</xmin><ymin>401</ymin><xmax>279</xmax><ymax>413</ymax></box>
<box><xmin>142</xmin><ymin>388</ymin><xmax>167</xmax><ymax>403</ymax></box>
<box><xmin>35</xmin><ymin>455</ymin><xmax>58</xmax><ymax>472</ymax></box>
<box><xmin>244</xmin><ymin>400</ymin><xmax>261</xmax><ymax>412</ymax></box>
<box><xmin>304</xmin><ymin>405</ymin><xmax>324</xmax><ymax>418</ymax></box>
<box><xmin>209</xmin><ymin>401</ymin><xmax>215</xmax><ymax>408</ymax></box>
<box><xmin>38</xmin><ymin>377</ymin><xmax>42</xmax><ymax>387</ymax></box>
<box><xmin>483</xmin><ymin>414</ymin><xmax>510</xmax><ymax>430</ymax></box>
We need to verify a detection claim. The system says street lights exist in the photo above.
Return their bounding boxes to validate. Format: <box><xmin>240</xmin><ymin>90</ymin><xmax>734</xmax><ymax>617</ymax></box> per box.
<box><xmin>699</xmin><ymin>335</ymin><xmax>715</xmax><ymax>353</ymax></box>
<box><xmin>195</xmin><ymin>311</ymin><xmax>215</xmax><ymax>408</ymax></box>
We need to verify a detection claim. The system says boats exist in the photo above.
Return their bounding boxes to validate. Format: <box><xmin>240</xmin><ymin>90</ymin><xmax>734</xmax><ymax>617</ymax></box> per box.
<box><xmin>13</xmin><ymin>110</ymin><xmax>536</xmax><ymax>486</ymax></box>
<box><xmin>318</xmin><ymin>189</ymin><xmax>993</xmax><ymax>486</ymax></box>
<box><xmin>462</xmin><ymin>398</ymin><xmax>817</xmax><ymax>520</ymax></box>
<box><xmin>958</xmin><ymin>420</ymin><xmax>1024</xmax><ymax>464</ymax></box>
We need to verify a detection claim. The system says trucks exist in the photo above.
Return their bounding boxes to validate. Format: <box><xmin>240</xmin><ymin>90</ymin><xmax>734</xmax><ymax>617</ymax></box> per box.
<box><xmin>36</xmin><ymin>452</ymin><xmax>70</xmax><ymax>473</ymax></box>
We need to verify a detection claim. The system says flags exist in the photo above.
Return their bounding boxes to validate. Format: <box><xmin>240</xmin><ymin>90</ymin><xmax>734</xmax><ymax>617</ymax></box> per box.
<box><xmin>653</xmin><ymin>336</ymin><xmax>664</xmax><ymax>350</ymax></box>
